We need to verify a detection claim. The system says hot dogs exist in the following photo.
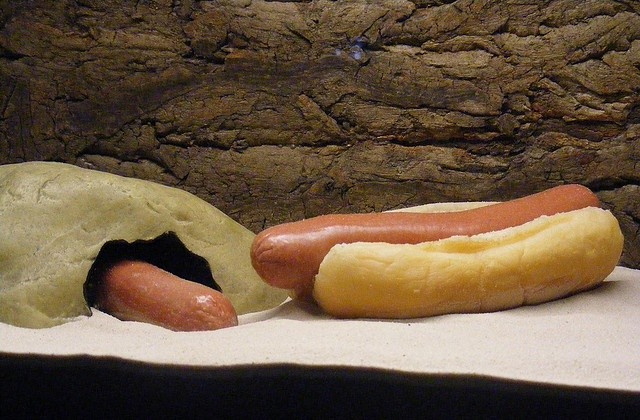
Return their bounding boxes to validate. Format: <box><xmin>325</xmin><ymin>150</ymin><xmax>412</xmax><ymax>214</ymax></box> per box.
<box><xmin>0</xmin><ymin>160</ymin><xmax>288</xmax><ymax>333</ymax></box>
<box><xmin>251</xmin><ymin>185</ymin><xmax>625</xmax><ymax>318</ymax></box>
